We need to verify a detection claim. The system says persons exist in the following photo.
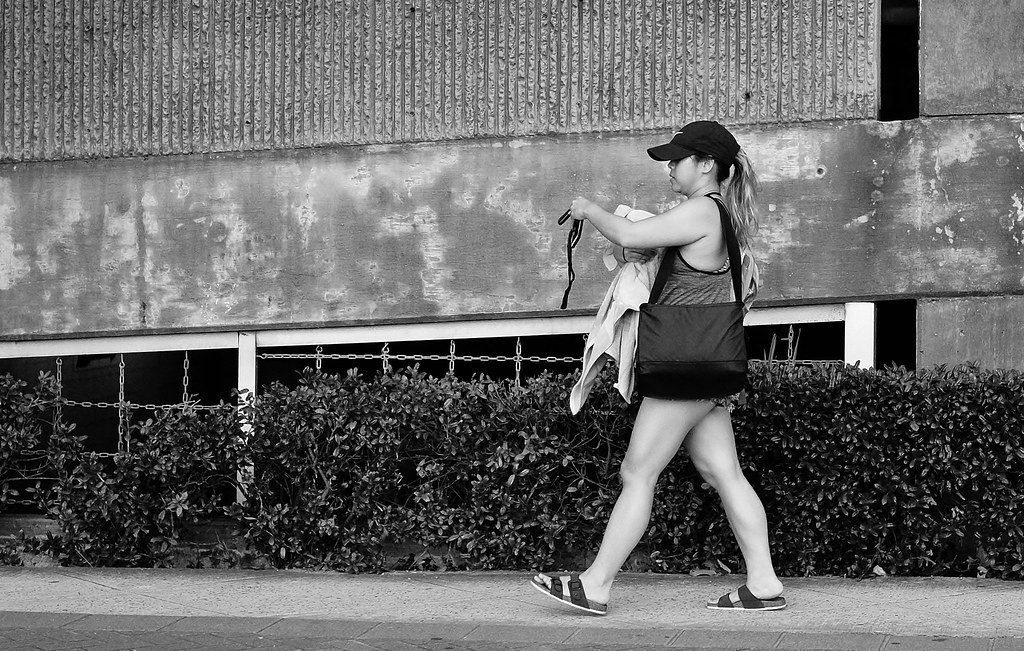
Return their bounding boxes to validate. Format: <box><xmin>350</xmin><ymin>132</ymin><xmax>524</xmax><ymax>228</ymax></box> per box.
<box><xmin>532</xmin><ymin>120</ymin><xmax>788</xmax><ymax>615</ymax></box>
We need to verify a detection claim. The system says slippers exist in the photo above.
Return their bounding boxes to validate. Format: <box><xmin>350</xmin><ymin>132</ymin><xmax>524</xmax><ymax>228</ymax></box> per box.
<box><xmin>530</xmin><ymin>571</ymin><xmax>607</xmax><ymax>615</ymax></box>
<box><xmin>706</xmin><ymin>584</ymin><xmax>787</xmax><ymax>611</ymax></box>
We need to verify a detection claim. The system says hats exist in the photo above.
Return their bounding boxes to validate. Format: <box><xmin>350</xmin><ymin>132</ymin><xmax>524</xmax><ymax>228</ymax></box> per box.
<box><xmin>646</xmin><ymin>120</ymin><xmax>740</xmax><ymax>167</ymax></box>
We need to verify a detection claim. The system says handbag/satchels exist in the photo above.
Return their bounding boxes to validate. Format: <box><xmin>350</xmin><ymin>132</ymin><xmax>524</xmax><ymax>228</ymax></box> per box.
<box><xmin>636</xmin><ymin>196</ymin><xmax>748</xmax><ymax>400</ymax></box>
